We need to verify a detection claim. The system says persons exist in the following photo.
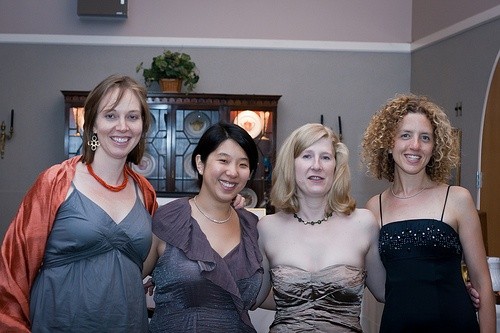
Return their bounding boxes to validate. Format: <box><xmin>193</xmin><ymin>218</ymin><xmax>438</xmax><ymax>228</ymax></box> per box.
<box><xmin>0</xmin><ymin>74</ymin><xmax>246</xmax><ymax>333</ymax></box>
<box><xmin>145</xmin><ymin>123</ymin><xmax>480</xmax><ymax>333</ymax></box>
<box><xmin>361</xmin><ymin>95</ymin><xmax>497</xmax><ymax>333</ymax></box>
<box><xmin>142</xmin><ymin>122</ymin><xmax>276</xmax><ymax>333</ymax></box>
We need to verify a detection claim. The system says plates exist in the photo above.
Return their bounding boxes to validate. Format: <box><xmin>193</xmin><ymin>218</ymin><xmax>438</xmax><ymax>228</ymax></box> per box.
<box><xmin>233</xmin><ymin>111</ymin><xmax>263</xmax><ymax>139</ymax></box>
<box><xmin>149</xmin><ymin>112</ymin><xmax>156</xmax><ymax>135</ymax></box>
<box><xmin>184</xmin><ymin>112</ymin><xmax>212</xmax><ymax>138</ymax></box>
<box><xmin>128</xmin><ymin>152</ymin><xmax>155</xmax><ymax>177</ymax></box>
<box><xmin>239</xmin><ymin>187</ymin><xmax>258</xmax><ymax>208</ymax></box>
<box><xmin>184</xmin><ymin>152</ymin><xmax>198</xmax><ymax>178</ymax></box>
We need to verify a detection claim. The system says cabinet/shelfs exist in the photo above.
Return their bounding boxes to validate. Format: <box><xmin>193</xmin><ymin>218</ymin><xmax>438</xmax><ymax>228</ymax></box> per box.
<box><xmin>60</xmin><ymin>90</ymin><xmax>283</xmax><ymax>215</ymax></box>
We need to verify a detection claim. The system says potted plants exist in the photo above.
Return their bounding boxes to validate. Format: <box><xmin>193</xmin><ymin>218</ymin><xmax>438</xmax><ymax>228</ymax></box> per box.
<box><xmin>135</xmin><ymin>50</ymin><xmax>200</xmax><ymax>94</ymax></box>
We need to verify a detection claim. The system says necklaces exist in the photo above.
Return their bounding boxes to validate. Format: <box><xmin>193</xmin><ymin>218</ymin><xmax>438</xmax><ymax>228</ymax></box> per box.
<box><xmin>87</xmin><ymin>161</ymin><xmax>128</xmax><ymax>192</ymax></box>
<box><xmin>390</xmin><ymin>181</ymin><xmax>432</xmax><ymax>199</ymax></box>
<box><xmin>292</xmin><ymin>209</ymin><xmax>334</xmax><ymax>226</ymax></box>
<box><xmin>194</xmin><ymin>195</ymin><xmax>234</xmax><ymax>223</ymax></box>
